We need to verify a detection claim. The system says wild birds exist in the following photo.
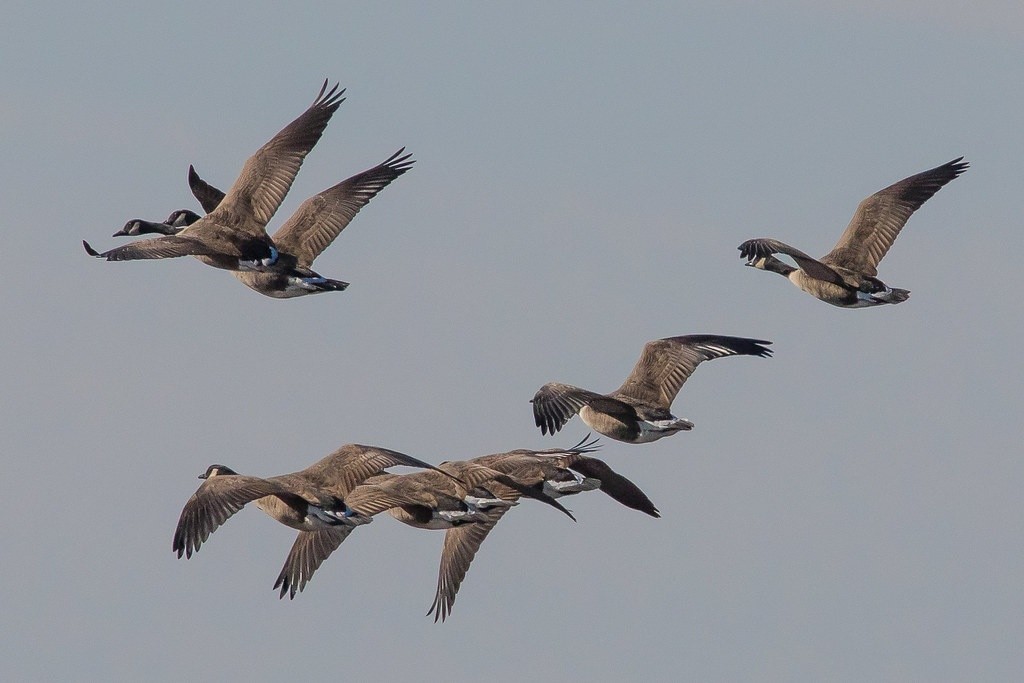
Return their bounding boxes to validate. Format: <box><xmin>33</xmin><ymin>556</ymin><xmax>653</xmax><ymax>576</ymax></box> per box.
<box><xmin>737</xmin><ymin>155</ymin><xmax>970</xmax><ymax>309</ymax></box>
<box><xmin>172</xmin><ymin>433</ymin><xmax>661</xmax><ymax>624</ymax></box>
<box><xmin>529</xmin><ymin>334</ymin><xmax>773</xmax><ymax>445</ymax></box>
<box><xmin>81</xmin><ymin>77</ymin><xmax>416</xmax><ymax>300</ymax></box>
